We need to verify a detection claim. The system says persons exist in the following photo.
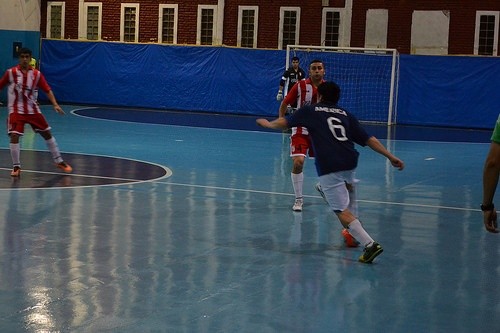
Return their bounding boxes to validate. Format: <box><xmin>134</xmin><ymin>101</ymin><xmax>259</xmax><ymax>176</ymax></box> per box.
<box><xmin>0</xmin><ymin>48</ymin><xmax>73</xmax><ymax>176</ymax></box>
<box><xmin>276</xmin><ymin>56</ymin><xmax>306</xmax><ymax>132</ymax></box>
<box><xmin>256</xmin><ymin>82</ymin><xmax>404</xmax><ymax>263</ymax></box>
<box><xmin>481</xmin><ymin>115</ymin><xmax>500</xmax><ymax>233</ymax></box>
<box><xmin>279</xmin><ymin>59</ymin><xmax>330</xmax><ymax>212</ymax></box>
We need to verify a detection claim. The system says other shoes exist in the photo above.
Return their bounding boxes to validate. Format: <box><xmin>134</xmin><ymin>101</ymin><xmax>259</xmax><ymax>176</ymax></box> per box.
<box><xmin>292</xmin><ymin>200</ymin><xmax>304</xmax><ymax>211</ymax></box>
<box><xmin>314</xmin><ymin>182</ymin><xmax>329</xmax><ymax>205</ymax></box>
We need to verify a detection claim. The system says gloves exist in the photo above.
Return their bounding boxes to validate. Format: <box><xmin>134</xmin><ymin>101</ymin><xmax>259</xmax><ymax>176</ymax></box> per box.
<box><xmin>276</xmin><ymin>90</ymin><xmax>284</xmax><ymax>100</ymax></box>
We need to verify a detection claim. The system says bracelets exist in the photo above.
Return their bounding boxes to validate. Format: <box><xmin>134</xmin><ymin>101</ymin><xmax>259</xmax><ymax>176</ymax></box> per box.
<box><xmin>480</xmin><ymin>202</ymin><xmax>494</xmax><ymax>211</ymax></box>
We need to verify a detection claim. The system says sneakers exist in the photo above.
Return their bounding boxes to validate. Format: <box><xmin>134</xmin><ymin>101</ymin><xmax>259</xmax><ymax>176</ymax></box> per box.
<box><xmin>358</xmin><ymin>242</ymin><xmax>384</xmax><ymax>263</ymax></box>
<box><xmin>343</xmin><ymin>236</ymin><xmax>359</xmax><ymax>245</ymax></box>
<box><xmin>11</xmin><ymin>167</ymin><xmax>20</xmax><ymax>177</ymax></box>
<box><xmin>55</xmin><ymin>161</ymin><xmax>73</xmax><ymax>173</ymax></box>
